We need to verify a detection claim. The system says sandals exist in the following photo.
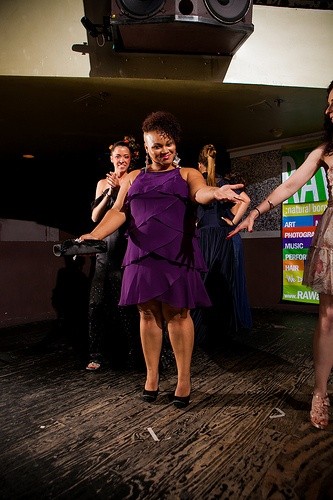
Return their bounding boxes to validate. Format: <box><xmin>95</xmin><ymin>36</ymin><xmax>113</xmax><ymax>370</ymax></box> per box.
<box><xmin>85</xmin><ymin>362</ymin><xmax>101</xmax><ymax>370</ymax></box>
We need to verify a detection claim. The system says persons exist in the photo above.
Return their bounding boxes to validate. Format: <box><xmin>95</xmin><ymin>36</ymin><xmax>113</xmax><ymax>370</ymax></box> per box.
<box><xmin>193</xmin><ymin>143</ymin><xmax>252</xmax><ymax>350</ymax></box>
<box><xmin>85</xmin><ymin>135</ymin><xmax>140</xmax><ymax>370</ymax></box>
<box><xmin>75</xmin><ymin>112</ymin><xmax>247</xmax><ymax>408</ymax></box>
<box><xmin>225</xmin><ymin>80</ymin><xmax>333</xmax><ymax>431</ymax></box>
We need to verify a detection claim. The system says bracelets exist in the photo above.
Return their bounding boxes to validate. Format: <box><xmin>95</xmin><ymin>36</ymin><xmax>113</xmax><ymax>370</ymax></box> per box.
<box><xmin>264</xmin><ymin>199</ymin><xmax>274</xmax><ymax>210</ymax></box>
<box><xmin>256</xmin><ymin>208</ymin><xmax>260</xmax><ymax>216</ymax></box>
<box><xmin>107</xmin><ymin>194</ymin><xmax>110</xmax><ymax>197</ymax></box>
<box><xmin>231</xmin><ymin>221</ymin><xmax>235</xmax><ymax>225</ymax></box>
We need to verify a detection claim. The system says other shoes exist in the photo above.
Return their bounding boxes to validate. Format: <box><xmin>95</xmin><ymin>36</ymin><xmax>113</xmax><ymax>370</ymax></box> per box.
<box><xmin>309</xmin><ymin>391</ymin><xmax>331</xmax><ymax>429</ymax></box>
<box><xmin>174</xmin><ymin>395</ymin><xmax>190</xmax><ymax>408</ymax></box>
<box><xmin>143</xmin><ymin>374</ymin><xmax>161</xmax><ymax>403</ymax></box>
